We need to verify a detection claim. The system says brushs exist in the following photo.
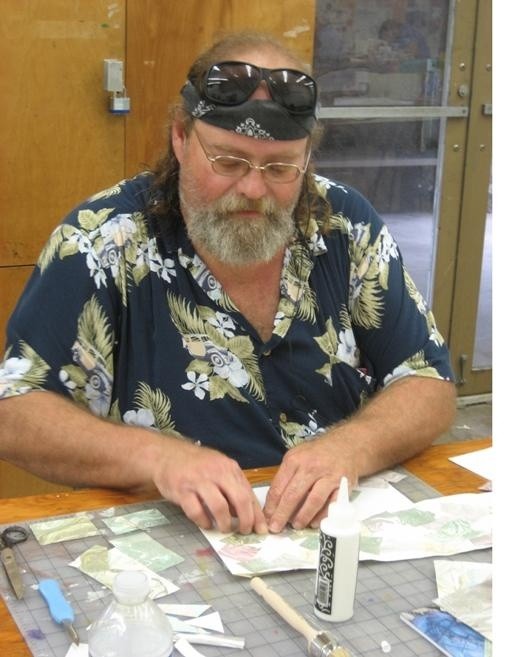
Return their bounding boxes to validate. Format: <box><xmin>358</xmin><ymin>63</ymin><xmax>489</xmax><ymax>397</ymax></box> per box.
<box><xmin>250</xmin><ymin>576</ymin><xmax>350</xmax><ymax>657</ymax></box>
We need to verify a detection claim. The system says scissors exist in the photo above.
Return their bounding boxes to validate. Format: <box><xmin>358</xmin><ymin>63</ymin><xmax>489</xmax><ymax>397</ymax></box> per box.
<box><xmin>0</xmin><ymin>525</ymin><xmax>29</xmax><ymax>599</ymax></box>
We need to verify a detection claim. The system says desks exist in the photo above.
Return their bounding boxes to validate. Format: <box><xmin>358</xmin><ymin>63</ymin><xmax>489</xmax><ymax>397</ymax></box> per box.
<box><xmin>2</xmin><ymin>437</ymin><xmax>495</xmax><ymax>657</ymax></box>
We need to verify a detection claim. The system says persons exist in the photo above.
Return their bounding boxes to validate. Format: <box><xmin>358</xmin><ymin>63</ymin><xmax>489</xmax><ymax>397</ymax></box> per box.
<box><xmin>0</xmin><ymin>34</ymin><xmax>458</xmax><ymax>537</ymax></box>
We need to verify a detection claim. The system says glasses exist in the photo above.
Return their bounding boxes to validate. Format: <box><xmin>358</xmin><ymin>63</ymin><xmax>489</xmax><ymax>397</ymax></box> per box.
<box><xmin>189</xmin><ymin>125</ymin><xmax>311</xmax><ymax>184</ymax></box>
<box><xmin>204</xmin><ymin>60</ymin><xmax>317</xmax><ymax>114</ymax></box>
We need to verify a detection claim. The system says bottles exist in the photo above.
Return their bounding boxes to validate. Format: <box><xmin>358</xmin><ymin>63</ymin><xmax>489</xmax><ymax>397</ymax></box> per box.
<box><xmin>313</xmin><ymin>475</ymin><xmax>364</xmax><ymax>624</ymax></box>
<box><xmin>86</xmin><ymin>566</ymin><xmax>177</xmax><ymax>657</ymax></box>
